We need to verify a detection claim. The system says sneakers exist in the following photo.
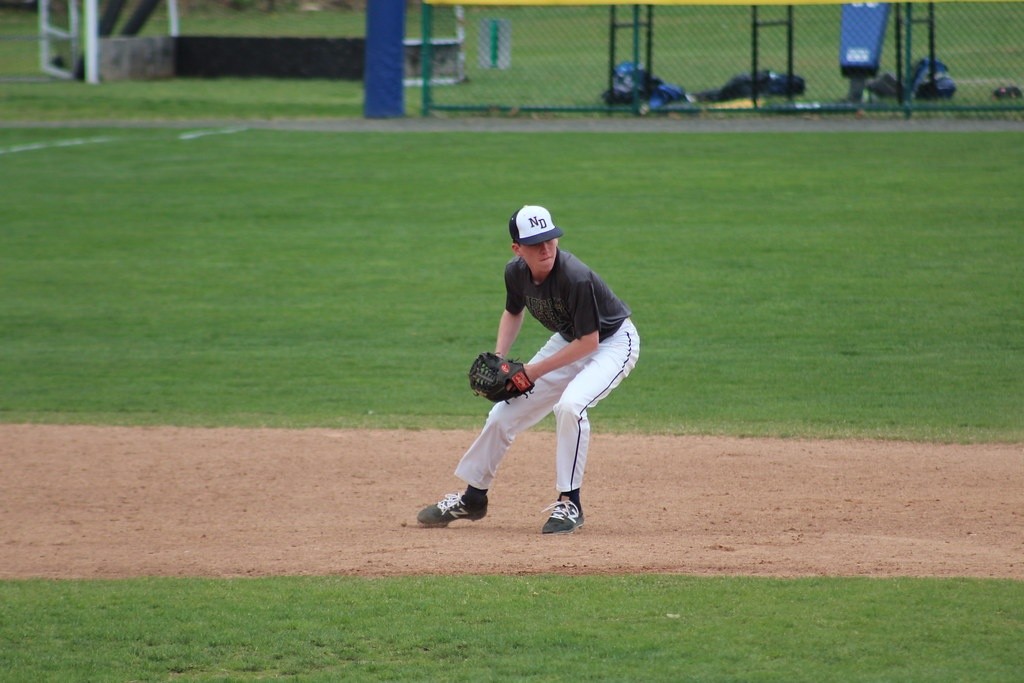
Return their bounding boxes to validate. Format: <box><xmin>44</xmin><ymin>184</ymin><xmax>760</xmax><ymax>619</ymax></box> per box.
<box><xmin>541</xmin><ymin>500</ymin><xmax>585</xmax><ymax>536</ymax></box>
<box><xmin>415</xmin><ymin>491</ymin><xmax>488</xmax><ymax>528</ymax></box>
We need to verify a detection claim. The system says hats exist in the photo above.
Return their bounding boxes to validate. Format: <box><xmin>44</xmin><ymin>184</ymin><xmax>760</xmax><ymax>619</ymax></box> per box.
<box><xmin>508</xmin><ymin>205</ymin><xmax>563</xmax><ymax>245</ymax></box>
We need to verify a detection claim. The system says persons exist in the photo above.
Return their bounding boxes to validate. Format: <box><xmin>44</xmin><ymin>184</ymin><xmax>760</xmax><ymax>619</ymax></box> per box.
<box><xmin>416</xmin><ymin>205</ymin><xmax>640</xmax><ymax>534</ymax></box>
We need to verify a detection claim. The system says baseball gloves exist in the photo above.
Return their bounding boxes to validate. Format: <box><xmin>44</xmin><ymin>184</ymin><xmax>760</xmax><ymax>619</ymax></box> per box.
<box><xmin>468</xmin><ymin>351</ymin><xmax>535</xmax><ymax>403</ymax></box>
